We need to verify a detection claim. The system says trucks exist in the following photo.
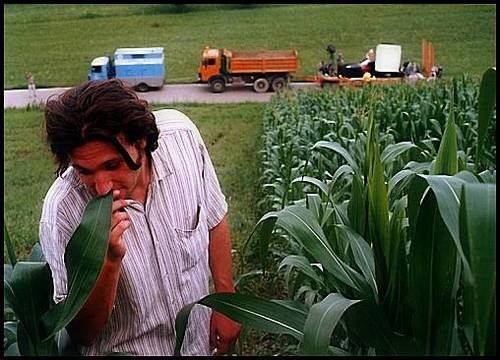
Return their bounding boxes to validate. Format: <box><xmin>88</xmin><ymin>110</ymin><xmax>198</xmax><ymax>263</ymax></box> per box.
<box><xmin>87</xmin><ymin>46</ymin><xmax>166</xmax><ymax>93</ymax></box>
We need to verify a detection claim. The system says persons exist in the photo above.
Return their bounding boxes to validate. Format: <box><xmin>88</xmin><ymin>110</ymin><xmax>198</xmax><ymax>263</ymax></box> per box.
<box><xmin>40</xmin><ymin>79</ymin><xmax>242</xmax><ymax>357</ymax></box>
<box><xmin>24</xmin><ymin>71</ymin><xmax>37</xmax><ymax>99</ymax></box>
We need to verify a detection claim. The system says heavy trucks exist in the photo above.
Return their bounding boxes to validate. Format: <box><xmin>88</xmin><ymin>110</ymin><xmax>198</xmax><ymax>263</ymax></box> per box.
<box><xmin>196</xmin><ymin>45</ymin><xmax>298</xmax><ymax>94</ymax></box>
